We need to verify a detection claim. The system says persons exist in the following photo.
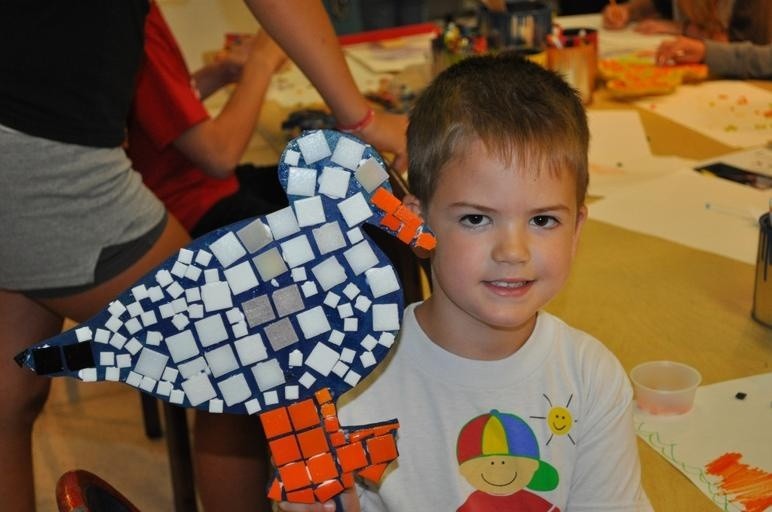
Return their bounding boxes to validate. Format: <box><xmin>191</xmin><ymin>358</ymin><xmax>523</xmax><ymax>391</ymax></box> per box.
<box><xmin>270</xmin><ymin>52</ymin><xmax>656</xmax><ymax>512</ymax></box>
<box><xmin>456</xmin><ymin>409</ymin><xmax>562</xmax><ymax>512</ymax></box>
<box><xmin>126</xmin><ymin>0</ymin><xmax>292</xmax><ymax>243</ymax></box>
<box><xmin>0</xmin><ymin>0</ymin><xmax>410</xmax><ymax>512</ymax></box>
<box><xmin>603</xmin><ymin>0</ymin><xmax>772</xmax><ymax>80</ymax></box>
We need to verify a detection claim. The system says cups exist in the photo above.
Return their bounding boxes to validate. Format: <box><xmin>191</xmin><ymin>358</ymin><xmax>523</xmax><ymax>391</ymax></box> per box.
<box><xmin>632</xmin><ymin>360</ymin><xmax>703</xmax><ymax>416</ymax></box>
<box><xmin>550</xmin><ymin>26</ymin><xmax>599</xmax><ymax>103</ymax></box>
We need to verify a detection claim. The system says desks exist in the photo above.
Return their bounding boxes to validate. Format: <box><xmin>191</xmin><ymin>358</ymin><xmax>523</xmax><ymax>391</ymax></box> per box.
<box><xmin>168</xmin><ymin>4</ymin><xmax>769</xmax><ymax>512</ymax></box>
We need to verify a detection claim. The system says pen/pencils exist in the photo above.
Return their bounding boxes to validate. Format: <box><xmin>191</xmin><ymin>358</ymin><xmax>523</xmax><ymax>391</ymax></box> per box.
<box><xmin>439</xmin><ymin>11</ymin><xmax>491</xmax><ymax>55</ymax></box>
<box><xmin>545</xmin><ymin>24</ymin><xmax>588</xmax><ymax>50</ymax></box>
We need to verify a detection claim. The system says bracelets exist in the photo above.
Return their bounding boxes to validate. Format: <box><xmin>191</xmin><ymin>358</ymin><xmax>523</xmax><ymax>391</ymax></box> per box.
<box><xmin>337</xmin><ymin>108</ymin><xmax>376</xmax><ymax>135</ymax></box>
